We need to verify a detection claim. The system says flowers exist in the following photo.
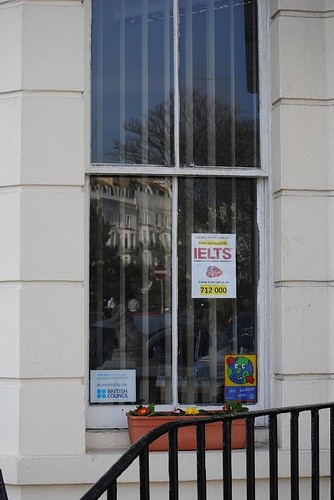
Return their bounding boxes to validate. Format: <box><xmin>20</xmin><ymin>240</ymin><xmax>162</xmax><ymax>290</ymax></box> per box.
<box><xmin>129</xmin><ymin>400</ymin><xmax>249</xmax><ymax>417</ymax></box>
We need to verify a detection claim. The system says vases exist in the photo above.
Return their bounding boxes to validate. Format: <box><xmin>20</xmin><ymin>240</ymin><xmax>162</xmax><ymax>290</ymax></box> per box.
<box><xmin>125</xmin><ymin>408</ymin><xmax>248</xmax><ymax>451</ymax></box>
